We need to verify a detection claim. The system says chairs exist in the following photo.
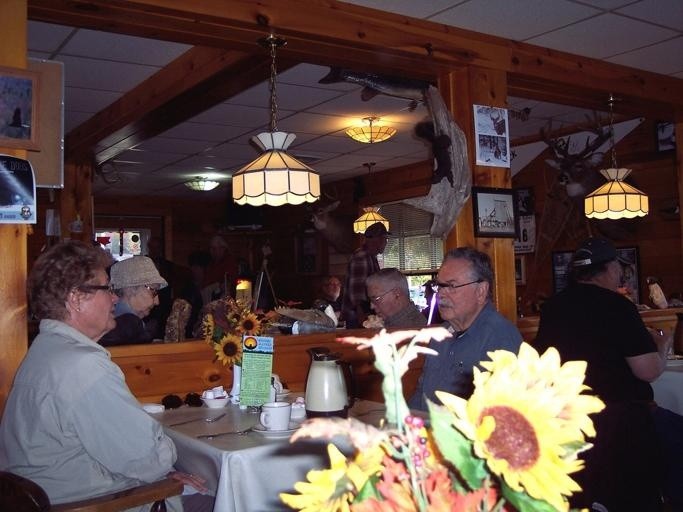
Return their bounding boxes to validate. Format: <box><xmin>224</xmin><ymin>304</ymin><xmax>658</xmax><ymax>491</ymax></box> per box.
<box><xmin>598</xmin><ymin>399</ymin><xmax>671</xmax><ymax>512</ymax></box>
<box><xmin>0</xmin><ymin>472</ymin><xmax>184</xmax><ymax>512</ymax></box>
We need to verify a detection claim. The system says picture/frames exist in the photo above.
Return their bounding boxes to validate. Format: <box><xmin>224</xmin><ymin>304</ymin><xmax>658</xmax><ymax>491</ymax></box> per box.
<box><xmin>551</xmin><ymin>246</ymin><xmax>641</xmax><ymax>304</ymax></box>
<box><xmin>515</xmin><ymin>255</ymin><xmax>526</xmax><ymax>286</ymax></box>
<box><xmin>655</xmin><ymin>120</ymin><xmax>675</xmax><ymax>154</ymax></box>
<box><xmin>515</xmin><ymin>187</ymin><xmax>532</xmax><ymax>215</ymax></box>
<box><xmin>0</xmin><ymin>66</ymin><xmax>41</xmax><ymax>151</ymax></box>
<box><xmin>472</xmin><ymin>187</ymin><xmax>520</xmax><ymax>237</ymax></box>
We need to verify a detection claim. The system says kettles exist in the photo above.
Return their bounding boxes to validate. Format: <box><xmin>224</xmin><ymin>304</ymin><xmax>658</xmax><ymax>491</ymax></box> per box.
<box><xmin>303</xmin><ymin>347</ymin><xmax>355</xmax><ymax>418</ymax></box>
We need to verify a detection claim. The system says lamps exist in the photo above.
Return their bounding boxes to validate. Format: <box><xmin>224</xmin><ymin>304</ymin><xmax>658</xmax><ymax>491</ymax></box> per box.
<box><xmin>353</xmin><ymin>162</ymin><xmax>390</xmax><ymax>234</ymax></box>
<box><xmin>231</xmin><ymin>35</ymin><xmax>322</xmax><ymax>208</ymax></box>
<box><xmin>345</xmin><ymin>117</ymin><xmax>397</xmax><ymax>144</ymax></box>
<box><xmin>584</xmin><ymin>93</ymin><xmax>649</xmax><ymax>221</ymax></box>
<box><xmin>184</xmin><ymin>176</ymin><xmax>220</xmax><ymax>191</ymax></box>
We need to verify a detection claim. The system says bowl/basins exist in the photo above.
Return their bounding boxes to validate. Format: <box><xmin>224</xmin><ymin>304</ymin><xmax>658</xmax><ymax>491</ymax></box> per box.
<box><xmin>202</xmin><ymin>397</ymin><xmax>229</xmax><ymax>407</ymax></box>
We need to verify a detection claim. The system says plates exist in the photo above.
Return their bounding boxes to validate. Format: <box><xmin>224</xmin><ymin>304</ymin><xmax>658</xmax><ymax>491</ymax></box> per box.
<box><xmin>251</xmin><ymin>421</ymin><xmax>301</xmax><ymax>437</ymax></box>
<box><xmin>275</xmin><ymin>389</ymin><xmax>291</xmax><ymax>399</ymax></box>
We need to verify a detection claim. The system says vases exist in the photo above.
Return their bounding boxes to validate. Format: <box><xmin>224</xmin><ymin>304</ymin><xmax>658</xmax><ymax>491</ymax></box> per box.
<box><xmin>230</xmin><ymin>364</ymin><xmax>242</xmax><ymax>404</ymax></box>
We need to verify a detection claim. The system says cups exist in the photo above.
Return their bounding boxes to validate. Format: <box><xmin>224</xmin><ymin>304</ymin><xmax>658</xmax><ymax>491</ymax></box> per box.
<box><xmin>272</xmin><ymin>372</ymin><xmax>283</xmax><ymax>394</ymax></box>
<box><xmin>260</xmin><ymin>402</ymin><xmax>291</xmax><ymax>429</ymax></box>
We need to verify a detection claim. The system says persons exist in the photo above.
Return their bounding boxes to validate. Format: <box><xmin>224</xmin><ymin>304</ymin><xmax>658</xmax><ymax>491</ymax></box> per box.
<box><xmin>534</xmin><ymin>233</ymin><xmax>681</xmax><ymax>507</ymax></box>
<box><xmin>420</xmin><ymin>280</ymin><xmax>443</xmax><ymax>323</ymax></box>
<box><xmin>0</xmin><ymin>239</ymin><xmax>217</xmax><ymax>511</ymax></box>
<box><xmin>315</xmin><ymin>276</ymin><xmax>354</xmax><ymax>321</ymax></box>
<box><xmin>366</xmin><ymin>268</ymin><xmax>425</xmax><ymax>329</ymax></box>
<box><xmin>144</xmin><ymin>236</ymin><xmax>177</xmax><ymax>338</ymax></box>
<box><xmin>340</xmin><ymin>223</ymin><xmax>392</xmax><ymax>329</ymax></box>
<box><xmin>100</xmin><ymin>254</ymin><xmax>169</xmax><ymax>346</ymax></box>
<box><xmin>409</xmin><ymin>247</ymin><xmax>523</xmax><ymax>412</ymax></box>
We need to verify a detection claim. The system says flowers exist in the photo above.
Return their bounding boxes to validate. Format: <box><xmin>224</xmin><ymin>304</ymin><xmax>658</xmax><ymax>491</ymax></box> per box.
<box><xmin>279</xmin><ymin>327</ymin><xmax>606</xmax><ymax>512</ymax></box>
<box><xmin>201</xmin><ymin>307</ymin><xmax>262</xmax><ymax>366</ymax></box>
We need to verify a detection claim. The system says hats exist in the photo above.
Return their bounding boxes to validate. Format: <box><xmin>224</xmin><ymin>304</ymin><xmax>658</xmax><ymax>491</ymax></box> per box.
<box><xmin>364</xmin><ymin>222</ymin><xmax>392</xmax><ymax>237</ymax></box>
<box><xmin>107</xmin><ymin>256</ymin><xmax>167</xmax><ymax>290</ymax></box>
<box><xmin>571</xmin><ymin>239</ymin><xmax>634</xmax><ymax>268</ymax></box>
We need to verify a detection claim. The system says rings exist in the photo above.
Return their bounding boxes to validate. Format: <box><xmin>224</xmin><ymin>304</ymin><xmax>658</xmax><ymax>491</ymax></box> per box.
<box><xmin>188</xmin><ymin>473</ymin><xmax>196</xmax><ymax>478</ymax></box>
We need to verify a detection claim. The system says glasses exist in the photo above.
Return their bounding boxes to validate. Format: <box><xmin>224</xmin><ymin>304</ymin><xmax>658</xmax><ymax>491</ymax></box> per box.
<box><xmin>370</xmin><ymin>288</ymin><xmax>392</xmax><ymax>304</ymax></box>
<box><xmin>78</xmin><ymin>282</ymin><xmax>122</xmax><ymax>297</ymax></box>
<box><xmin>143</xmin><ymin>285</ymin><xmax>159</xmax><ymax>298</ymax></box>
<box><xmin>161</xmin><ymin>392</ymin><xmax>203</xmax><ymax>410</ymax></box>
<box><xmin>428</xmin><ymin>280</ymin><xmax>483</xmax><ymax>293</ymax></box>
